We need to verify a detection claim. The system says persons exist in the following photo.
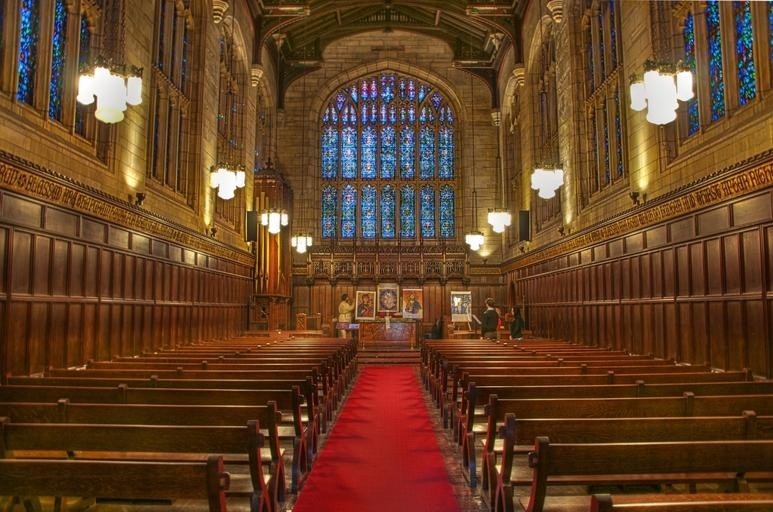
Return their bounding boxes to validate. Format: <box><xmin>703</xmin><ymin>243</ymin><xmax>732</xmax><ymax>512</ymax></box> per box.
<box><xmin>451</xmin><ymin>296</ymin><xmax>466</xmax><ymax>314</ymax></box>
<box><xmin>337</xmin><ymin>294</ymin><xmax>355</xmax><ymax>339</ymax></box>
<box><xmin>357</xmin><ymin>294</ymin><xmax>373</xmax><ymax>317</ymax></box>
<box><xmin>494</xmin><ymin>305</ymin><xmax>525</xmax><ymax>342</ymax></box>
<box><xmin>404</xmin><ymin>291</ymin><xmax>422</xmax><ymax>314</ymax></box>
<box><xmin>479</xmin><ymin>298</ymin><xmax>499</xmax><ymax>341</ymax></box>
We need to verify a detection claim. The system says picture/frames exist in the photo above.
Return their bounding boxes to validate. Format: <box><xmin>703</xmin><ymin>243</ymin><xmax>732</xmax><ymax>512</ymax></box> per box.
<box><xmin>402</xmin><ymin>289</ymin><xmax>424</xmax><ymax>320</ymax></box>
<box><xmin>376</xmin><ymin>282</ymin><xmax>400</xmax><ymax>315</ymax></box>
<box><xmin>354</xmin><ymin>290</ymin><xmax>377</xmax><ymax>321</ymax></box>
<box><xmin>450</xmin><ymin>290</ymin><xmax>472</xmax><ymax>322</ymax></box>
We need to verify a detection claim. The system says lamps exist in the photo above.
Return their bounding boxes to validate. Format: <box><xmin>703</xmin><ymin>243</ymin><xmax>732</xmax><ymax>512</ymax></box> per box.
<box><xmin>486</xmin><ymin>36</ymin><xmax>512</xmax><ymax>234</ymax></box>
<box><xmin>289</xmin><ymin>0</ymin><xmax>314</xmax><ymax>255</ymax></box>
<box><xmin>465</xmin><ymin>5</ymin><xmax>484</xmax><ymax>251</ymax></box>
<box><xmin>530</xmin><ymin>2</ymin><xmax>563</xmax><ymax>199</ymax></box>
<box><xmin>209</xmin><ymin>1</ymin><xmax>247</xmax><ymax>199</ymax></box>
<box><xmin>76</xmin><ymin>0</ymin><xmax>144</xmax><ymax>125</ymax></box>
<box><xmin>259</xmin><ymin>0</ymin><xmax>289</xmax><ymax>234</ymax></box>
<box><xmin>629</xmin><ymin>53</ymin><xmax>694</xmax><ymax>126</ymax></box>
